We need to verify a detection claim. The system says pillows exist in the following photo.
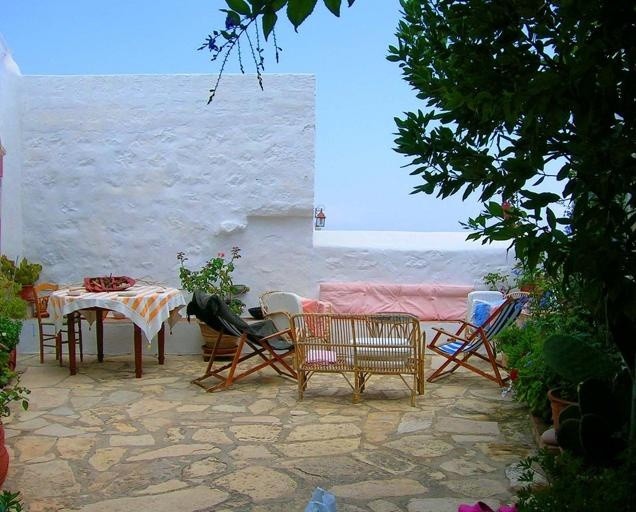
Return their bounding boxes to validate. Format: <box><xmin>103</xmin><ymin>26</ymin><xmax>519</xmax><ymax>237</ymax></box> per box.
<box><xmin>301</xmin><ymin>301</ymin><xmax>325</xmax><ymax>336</ymax></box>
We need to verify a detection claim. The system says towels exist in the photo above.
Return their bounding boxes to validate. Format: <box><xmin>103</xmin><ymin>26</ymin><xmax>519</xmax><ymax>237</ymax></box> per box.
<box><xmin>306</xmin><ymin>350</ymin><xmax>337</xmax><ymax>366</ymax></box>
<box><xmin>472</xmin><ymin>303</ymin><xmax>490</xmax><ymax>328</ymax></box>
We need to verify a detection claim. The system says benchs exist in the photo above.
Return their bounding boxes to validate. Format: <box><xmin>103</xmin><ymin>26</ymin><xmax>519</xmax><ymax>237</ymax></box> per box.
<box><xmin>318</xmin><ymin>282</ymin><xmax>475</xmax><ymax>355</ymax></box>
<box><xmin>286</xmin><ymin>312</ymin><xmax>426</xmax><ymax>406</ymax></box>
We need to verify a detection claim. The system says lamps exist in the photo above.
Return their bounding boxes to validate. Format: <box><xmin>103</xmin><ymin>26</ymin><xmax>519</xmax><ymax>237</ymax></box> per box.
<box><xmin>314</xmin><ymin>207</ymin><xmax>326</xmax><ymax>227</ymax></box>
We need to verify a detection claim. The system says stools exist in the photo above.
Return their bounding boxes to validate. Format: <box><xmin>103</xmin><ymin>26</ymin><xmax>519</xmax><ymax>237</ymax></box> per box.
<box><xmin>8</xmin><ymin>312</ymin><xmax>62</xmax><ymax>373</ymax></box>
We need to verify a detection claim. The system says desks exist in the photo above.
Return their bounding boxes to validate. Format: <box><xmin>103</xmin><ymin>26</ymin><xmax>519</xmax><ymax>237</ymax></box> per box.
<box><xmin>46</xmin><ymin>287</ymin><xmax>186</xmax><ymax>377</ymax></box>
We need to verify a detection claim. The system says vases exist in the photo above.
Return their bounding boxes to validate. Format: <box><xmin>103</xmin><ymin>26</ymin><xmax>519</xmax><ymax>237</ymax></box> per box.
<box><xmin>196</xmin><ymin>318</ymin><xmax>241</xmax><ymax>348</ymax></box>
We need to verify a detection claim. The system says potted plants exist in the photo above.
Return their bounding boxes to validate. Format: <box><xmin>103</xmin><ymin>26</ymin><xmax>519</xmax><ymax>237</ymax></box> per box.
<box><xmin>0</xmin><ymin>275</ymin><xmax>31</xmax><ymax>485</ymax></box>
<box><xmin>548</xmin><ymin>332</ymin><xmax>633</xmax><ymax>456</ymax></box>
<box><xmin>0</xmin><ymin>254</ymin><xmax>42</xmax><ymax>301</ymax></box>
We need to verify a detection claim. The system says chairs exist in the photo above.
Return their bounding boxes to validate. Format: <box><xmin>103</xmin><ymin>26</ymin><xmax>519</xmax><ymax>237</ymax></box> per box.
<box><xmin>261</xmin><ymin>291</ymin><xmax>334</xmax><ymax>368</ymax></box>
<box><xmin>426</xmin><ymin>295</ymin><xmax>530</xmax><ymax>387</ymax></box>
<box><xmin>189</xmin><ymin>289</ymin><xmax>297</xmax><ymax>393</ymax></box>
<box><xmin>33</xmin><ymin>282</ymin><xmax>82</xmax><ymax>368</ymax></box>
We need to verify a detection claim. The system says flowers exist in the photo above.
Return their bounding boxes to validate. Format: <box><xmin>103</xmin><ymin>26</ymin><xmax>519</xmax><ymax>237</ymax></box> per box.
<box><xmin>177</xmin><ymin>246</ymin><xmax>250</xmax><ymax>317</ymax></box>
